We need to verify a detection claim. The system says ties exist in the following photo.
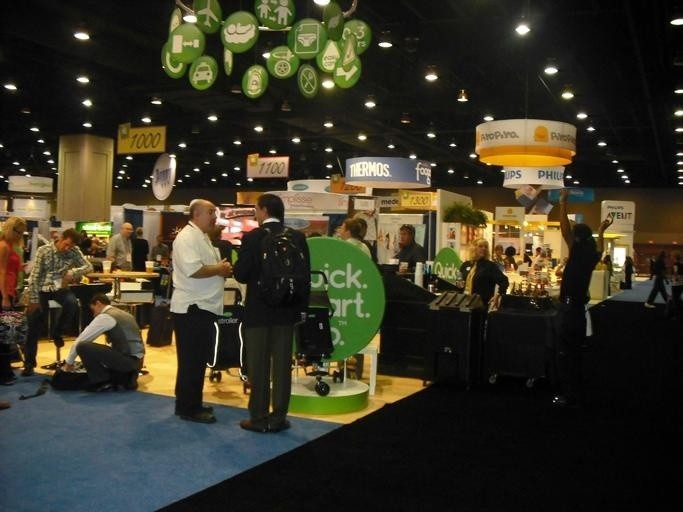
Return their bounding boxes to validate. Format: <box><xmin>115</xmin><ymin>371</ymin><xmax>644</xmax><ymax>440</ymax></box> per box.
<box><xmin>464</xmin><ymin>263</ymin><xmax>477</xmax><ymax>295</ymax></box>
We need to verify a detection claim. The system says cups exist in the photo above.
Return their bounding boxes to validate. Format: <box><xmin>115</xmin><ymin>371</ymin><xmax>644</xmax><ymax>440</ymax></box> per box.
<box><xmin>103</xmin><ymin>261</ymin><xmax>111</xmax><ymax>273</ymax></box>
<box><xmin>415</xmin><ymin>262</ymin><xmax>423</xmax><ymax>287</ymax></box>
<box><xmin>145</xmin><ymin>261</ymin><xmax>154</xmax><ymax>274</ymax></box>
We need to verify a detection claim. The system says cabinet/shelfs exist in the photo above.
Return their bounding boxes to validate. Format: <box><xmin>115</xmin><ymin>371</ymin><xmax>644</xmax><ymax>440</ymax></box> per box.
<box><xmin>482</xmin><ymin>293</ymin><xmax>563</xmax><ymax>391</ymax></box>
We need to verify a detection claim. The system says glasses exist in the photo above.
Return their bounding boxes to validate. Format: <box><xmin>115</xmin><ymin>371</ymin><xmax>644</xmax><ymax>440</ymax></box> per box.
<box><xmin>12</xmin><ymin>228</ymin><xmax>22</xmax><ymax>234</ymax></box>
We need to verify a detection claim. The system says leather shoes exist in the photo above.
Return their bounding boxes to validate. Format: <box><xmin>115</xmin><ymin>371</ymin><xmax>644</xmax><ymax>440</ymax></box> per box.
<box><xmin>1</xmin><ymin>371</ymin><xmax>13</xmax><ymax>376</ymax></box>
<box><xmin>127</xmin><ymin>372</ymin><xmax>137</xmax><ymax>391</ymax></box>
<box><xmin>267</xmin><ymin>420</ymin><xmax>291</xmax><ymax>433</ymax></box>
<box><xmin>240</xmin><ymin>420</ymin><xmax>267</xmax><ymax>433</ymax></box>
<box><xmin>1</xmin><ymin>379</ymin><xmax>13</xmax><ymax>383</ymax></box>
<box><xmin>182</xmin><ymin>412</ymin><xmax>215</xmax><ymax>422</ymax></box>
<box><xmin>197</xmin><ymin>404</ymin><xmax>215</xmax><ymax>412</ymax></box>
<box><xmin>94</xmin><ymin>384</ymin><xmax>115</xmax><ymax>391</ymax></box>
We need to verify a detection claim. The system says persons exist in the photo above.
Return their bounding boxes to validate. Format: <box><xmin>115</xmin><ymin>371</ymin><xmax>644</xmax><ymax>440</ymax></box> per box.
<box><xmin>354</xmin><ymin>218</ymin><xmax>377</xmax><ymax>262</ymax></box>
<box><xmin>491</xmin><ymin>242</ymin><xmax>554</xmax><ymax>274</ymax></box>
<box><xmin>0</xmin><ymin>216</ymin><xmax>28</xmax><ymax>380</ymax></box>
<box><xmin>340</xmin><ymin>218</ymin><xmax>373</xmax><ymax>378</ymax></box>
<box><xmin>80</xmin><ymin>229</ymin><xmax>107</xmax><ymax>257</ymax></box>
<box><xmin>133</xmin><ymin>227</ymin><xmax>149</xmax><ymax>272</ymax></box>
<box><xmin>455</xmin><ymin>238</ymin><xmax>509</xmax><ymax>366</ymax></box>
<box><xmin>170</xmin><ymin>199</ymin><xmax>233</xmax><ymax>423</ymax></box>
<box><xmin>209</xmin><ymin>226</ymin><xmax>233</xmax><ymax>265</ymax></box>
<box><xmin>234</xmin><ymin>195</ymin><xmax>310</xmax><ymax>432</ymax></box>
<box><xmin>105</xmin><ymin>222</ymin><xmax>134</xmax><ymax>271</ymax></box>
<box><xmin>550</xmin><ymin>190</ymin><xmax>613</xmax><ymax>408</ymax></box>
<box><xmin>151</xmin><ymin>234</ymin><xmax>169</xmax><ymax>260</ymax></box>
<box><xmin>22</xmin><ymin>229</ymin><xmax>93</xmax><ymax>375</ymax></box>
<box><xmin>393</xmin><ymin>224</ymin><xmax>427</xmax><ymax>272</ymax></box>
<box><xmin>603</xmin><ymin>250</ymin><xmax>681</xmax><ymax>309</ymax></box>
<box><xmin>22</xmin><ymin>226</ymin><xmax>59</xmax><ymax>255</ymax></box>
<box><xmin>61</xmin><ymin>293</ymin><xmax>145</xmax><ymax>392</ymax></box>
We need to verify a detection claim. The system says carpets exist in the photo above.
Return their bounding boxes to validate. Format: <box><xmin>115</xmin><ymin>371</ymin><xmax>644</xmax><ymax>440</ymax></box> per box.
<box><xmin>609</xmin><ymin>278</ymin><xmax>683</xmax><ymax>304</ymax></box>
<box><xmin>0</xmin><ymin>368</ymin><xmax>344</xmax><ymax>512</ymax></box>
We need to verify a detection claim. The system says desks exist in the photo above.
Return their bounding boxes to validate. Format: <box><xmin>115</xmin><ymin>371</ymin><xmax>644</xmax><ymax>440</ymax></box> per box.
<box><xmin>83</xmin><ymin>270</ymin><xmax>161</xmax><ymax>298</ymax></box>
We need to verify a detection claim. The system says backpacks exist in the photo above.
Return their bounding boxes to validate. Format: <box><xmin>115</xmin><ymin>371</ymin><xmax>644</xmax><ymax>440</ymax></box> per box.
<box><xmin>257</xmin><ymin>232</ymin><xmax>310</xmax><ymax>308</ymax></box>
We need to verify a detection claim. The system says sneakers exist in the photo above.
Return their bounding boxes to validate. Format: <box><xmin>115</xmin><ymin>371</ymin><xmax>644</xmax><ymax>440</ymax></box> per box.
<box><xmin>55</xmin><ymin>334</ymin><xmax>62</xmax><ymax>348</ymax></box>
<box><xmin>666</xmin><ymin>296</ymin><xmax>672</xmax><ymax>301</ymax></box>
<box><xmin>22</xmin><ymin>368</ymin><xmax>34</xmax><ymax>375</ymax></box>
<box><xmin>643</xmin><ymin>304</ymin><xmax>657</xmax><ymax>308</ymax></box>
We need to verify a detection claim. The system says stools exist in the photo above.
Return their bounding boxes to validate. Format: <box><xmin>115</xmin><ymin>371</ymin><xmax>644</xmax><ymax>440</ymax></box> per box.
<box><xmin>41</xmin><ymin>297</ymin><xmax>84</xmax><ymax>371</ymax></box>
